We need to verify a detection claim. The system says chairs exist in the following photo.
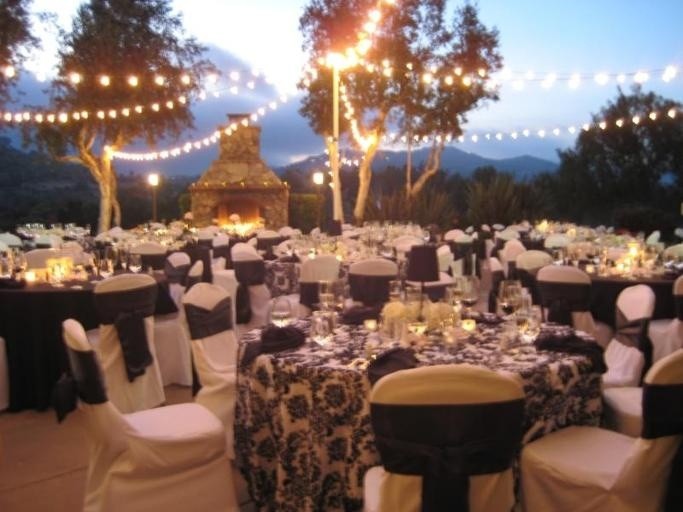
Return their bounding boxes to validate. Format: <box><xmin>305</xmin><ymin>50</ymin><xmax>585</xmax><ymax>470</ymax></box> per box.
<box><xmin>350</xmin><ymin>259</ymin><xmax>398</xmax><ymax>300</ymax></box>
<box><xmin>310</xmin><ymin>227</ymin><xmax>320</xmax><ymax>236</ymax></box>
<box><xmin>437</xmin><ymin>245</ymin><xmax>453</xmax><ymax>275</ymax></box>
<box><xmin>606</xmin><ymin>226</ymin><xmax>614</xmax><ymax>233</ymax></box>
<box><xmin>544</xmin><ymin>233</ymin><xmax>568</xmax><ymax>257</ymax></box>
<box><xmin>232</xmin><ymin>242</ymin><xmax>270</xmax><ymax>333</ymax></box>
<box><xmin>55</xmin><ymin>318</ymin><xmax>239</xmax><ymax>512</ymax></box>
<box><xmin>648</xmin><ymin>276</ymin><xmax>683</xmax><ymax>365</ymax></box>
<box><xmin>279</xmin><ymin>227</ymin><xmax>293</xmax><ymax>242</ymax></box>
<box><xmin>514</xmin><ymin>250</ymin><xmax>553</xmax><ymax>304</ymax></box>
<box><xmin>648</xmin><ymin>230</ymin><xmax>661</xmax><ymax>244</ymax></box>
<box><xmin>186</xmin><ymin>261</ymin><xmax>205</xmax><ymax>287</ymax></box>
<box><xmin>601</xmin><ymin>284</ymin><xmax>655</xmax><ymax>388</ymax></box>
<box><xmin>481</xmin><ymin>224</ymin><xmax>494</xmax><ymax>257</ymax></box>
<box><xmin>666</xmin><ymin>228</ymin><xmax>683</xmax><ymax>244</ymax></box>
<box><xmin>0</xmin><ymin>336</ymin><xmax>10</xmax><ymax>410</ymax></box>
<box><xmin>197</xmin><ymin>226</ymin><xmax>218</xmax><ymax>257</ymax></box>
<box><xmin>36</xmin><ymin>235</ymin><xmax>62</xmax><ymax>249</ymax></box>
<box><xmin>466</xmin><ymin>225</ymin><xmax>474</xmax><ymax>235</ymax></box>
<box><xmin>504</xmin><ymin>240</ymin><xmax>525</xmax><ymax>277</ymax></box>
<box><xmin>94</xmin><ymin>232</ymin><xmax>111</xmax><ymax>257</ymax></box>
<box><xmin>131</xmin><ymin>242</ymin><xmax>163</xmax><ymax>272</ymax></box>
<box><xmin>663</xmin><ymin>244</ymin><xmax>683</xmax><ymax>262</ymax></box>
<box><xmin>212</xmin><ymin>233</ymin><xmax>230</xmax><ymax>270</ymax></box>
<box><xmin>84</xmin><ymin>275</ymin><xmax>165</xmax><ymax>414</ymax></box>
<box><xmin>422</xmin><ymin>273</ymin><xmax>456</xmax><ymax>303</ymax></box>
<box><xmin>583</xmin><ymin>230</ymin><xmax>596</xmax><ymax>241</ymax></box>
<box><xmin>490</xmin><ymin>222</ymin><xmax>504</xmax><ymax>233</ymax></box>
<box><xmin>155</xmin><ymin>253</ymin><xmax>192</xmax><ymax>386</ymax></box>
<box><xmin>184</xmin><ymin>281</ymin><xmax>237</xmax><ymax>459</ymax></box>
<box><xmin>229</xmin><ymin>214</ymin><xmax>241</xmax><ymax>225</ymax></box>
<box><xmin>442</xmin><ymin>230</ymin><xmax>464</xmax><ymax>253</ymax></box>
<box><xmin>0</xmin><ymin>242</ymin><xmax>12</xmax><ymax>257</ymax></box>
<box><xmin>451</xmin><ymin>235</ymin><xmax>477</xmax><ymax>278</ymax></box>
<box><xmin>394</xmin><ymin>234</ymin><xmax>425</xmax><ymax>266</ymax></box>
<box><xmin>537</xmin><ymin>265</ymin><xmax>593</xmax><ymax>324</ymax></box>
<box><xmin>521</xmin><ymin>350</ymin><xmax>683</xmax><ymax>511</ymax></box>
<box><xmin>108</xmin><ymin>228</ymin><xmax>121</xmax><ymax>242</ymax></box>
<box><xmin>0</xmin><ymin>232</ymin><xmax>23</xmax><ymax>251</ymax></box>
<box><xmin>364</xmin><ymin>363</ymin><xmax>526</xmax><ymax>512</ymax></box>
<box><xmin>273</xmin><ymin>255</ymin><xmax>341</xmax><ymax>321</ymax></box>
<box><xmin>184</xmin><ymin>212</ymin><xmax>194</xmax><ymax>226</ymax></box>
<box><xmin>488</xmin><ymin>256</ymin><xmax>506</xmax><ymax>312</ymax></box>
<box><xmin>257</xmin><ymin>230</ymin><xmax>280</xmax><ymax>250</ymax></box>
<box><xmin>294</xmin><ymin>228</ymin><xmax>302</xmax><ymax>239</ymax></box>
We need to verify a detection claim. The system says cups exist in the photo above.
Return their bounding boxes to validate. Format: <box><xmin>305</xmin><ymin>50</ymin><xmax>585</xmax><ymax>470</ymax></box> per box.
<box><xmin>499</xmin><ymin>278</ymin><xmax>524</xmax><ymax>319</ymax></box>
<box><xmin>515</xmin><ymin>313</ymin><xmax>539</xmax><ymax>362</ymax></box>
<box><xmin>384</xmin><ymin>317</ymin><xmax>404</xmax><ymax>347</ymax></box>
<box><xmin>128</xmin><ymin>253</ymin><xmax>141</xmax><ymax>273</ymax></box>
<box><xmin>51</xmin><ymin>262</ymin><xmax>65</xmax><ymax>287</ymax></box>
<box><xmin>313</xmin><ymin>309</ymin><xmax>337</xmax><ymax>358</ymax></box>
<box><xmin>116</xmin><ymin>247</ymin><xmax>128</xmax><ymax>272</ymax></box>
<box><xmin>25</xmin><ymin>222</ymin><xmax>91</xmax><ymax>239</ymax></box>
<box><xmin>12</xmin><ymin>251</ymin><xmax>28</xmax><ymax>283</ymax></box>
<box><xmin>106</xmin><ymin>245</ymin><xmax>118</xmax><ymax>274</ymax></box>
<box><xmin>341</xmin><ymin>226</ymin><xmax>341</xmax><ymax>232</ymax></box>
<box><xmin>390</xmin><ymin>281</ymin><xmax>402</xmax><ymax>301</ymax></box>
<box><xmin>318</xmin><ymin>279</ymin><xmax>344</xmax><ymax>327</ymax></box>
<box><xmin>456</xmin><ymin>275</ymin><xmax>480</xmax><ymax>318</ymax></box>
<box><xmin>268</xmin><ymin>297</ymin><xmax>293</xmax><ymax>328</ymax></box>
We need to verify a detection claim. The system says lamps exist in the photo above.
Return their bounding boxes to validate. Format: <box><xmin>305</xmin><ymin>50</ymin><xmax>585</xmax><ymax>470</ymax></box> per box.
<box><xmin>405</xmin><ymin>245</ymin><xmax>441</xmax><ymax>323</ymax></box>
<box><xmin>319</xmin><ymin>217</ymin><xmax>330</xmax><ymax>231</ymax></box>
<box><xmin>326</xmin><ymin>219</ymin><xmax>343</xmax><ymax>251</ymax></box>
